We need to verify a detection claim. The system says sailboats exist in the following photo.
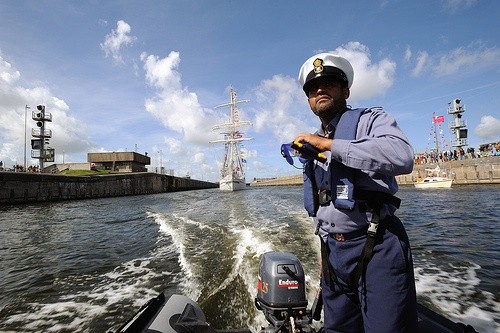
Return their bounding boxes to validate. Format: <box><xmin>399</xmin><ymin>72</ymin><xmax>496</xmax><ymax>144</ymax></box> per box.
<box><xmin>208</xmin><ymin>85</ymin><xmax>254</xmax><ymax>191</ymax></box>
<box><xmin>411</xmin><ymin>111</ymin><xmax>454</xmax><ymax>189</ymax></box>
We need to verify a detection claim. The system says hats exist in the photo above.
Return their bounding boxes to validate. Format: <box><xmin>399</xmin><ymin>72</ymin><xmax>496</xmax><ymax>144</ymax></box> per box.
<box><xmin>298</xmin><ymin>53</ymin><xmax>354</xmax><ymax>98</ymax></box>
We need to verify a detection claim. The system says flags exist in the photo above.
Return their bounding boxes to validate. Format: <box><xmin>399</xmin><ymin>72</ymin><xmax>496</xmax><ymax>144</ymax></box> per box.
<box><xmin>434</xmin><ymin>115</ymin><xmax>444</xmax><ymax>123</ymax></box>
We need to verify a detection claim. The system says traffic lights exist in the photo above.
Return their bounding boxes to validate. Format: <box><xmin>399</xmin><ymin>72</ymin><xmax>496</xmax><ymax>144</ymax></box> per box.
<box><xmin>36</xmin><ymin>105</ymin><xmax>45</xmax><ymax>128</ymax></box>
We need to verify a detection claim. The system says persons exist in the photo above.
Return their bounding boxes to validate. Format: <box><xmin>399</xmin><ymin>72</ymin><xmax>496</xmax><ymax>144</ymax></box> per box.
<box><xmin>13</xmin><ymin>164</ymin><xmax>40</xmax><ymax>173</ymax></box>
<box><xmin>413</xmin><ymin>139</ymin><xmax>500</xmax><ymax>165</ymax></box>
<box><xmin>0</xmin><ymin>161</ymin><xmax>3</xmax><ymax>171</ymax></box>
<box><xmin>292</xmin><ymin>53</ymin><xmax>418</xmax><ymax>333</ymax></box>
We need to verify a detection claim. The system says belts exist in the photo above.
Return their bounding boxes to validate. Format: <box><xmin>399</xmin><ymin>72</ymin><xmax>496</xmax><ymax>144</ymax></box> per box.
<box><xmin>328</xmin><ymin>215</ymin><xmax>396</xmax><ymax>241</ymax></box>
